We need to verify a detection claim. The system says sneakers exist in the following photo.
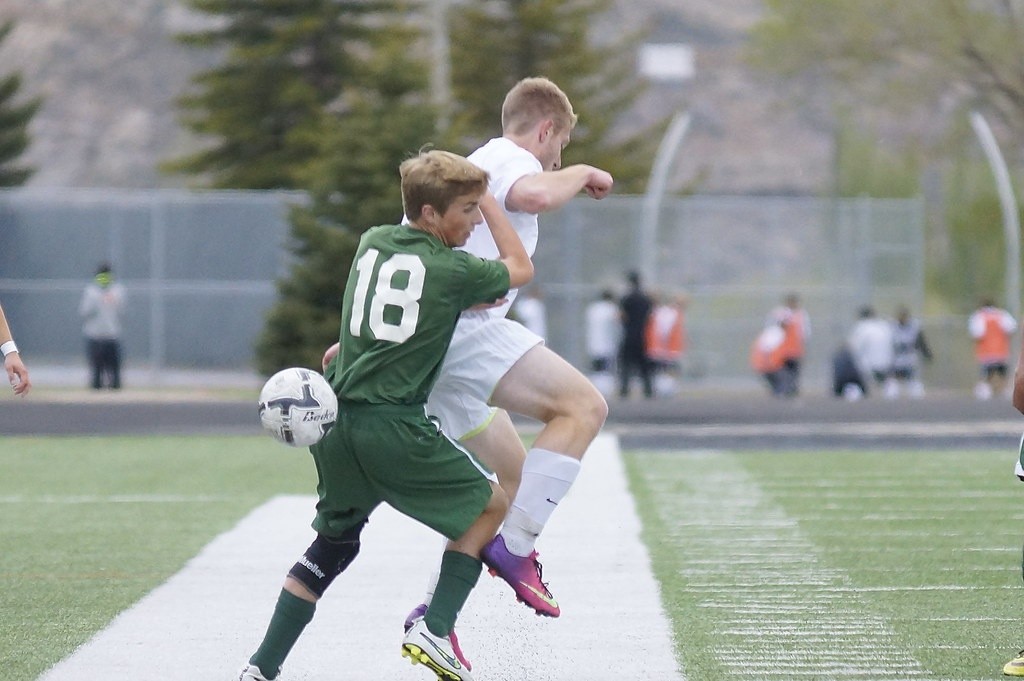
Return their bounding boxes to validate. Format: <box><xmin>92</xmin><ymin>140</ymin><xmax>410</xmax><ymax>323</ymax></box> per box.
<box><xmin>399</xmin><ymin>620</ymin><xmax>473</xmax><ymax>681</ymax></box>
<box><xmin>479</xmin><ymin>534</ymin><xmax>560</xmax><ymax>618</ymax></box>
<box><xmin>1003</xmin><ymin>650</ymin><xmax>1024</xmax><ymax>676</ymax></box>
<box><xmin>238</xmin><ymin>664</ymin><xmax>281</xmax><ymax>681</ymax></box>
<box><xmin>404</xmin><ymin>602</ymin><xmax>428</xmax><ymax>635</ymax></box>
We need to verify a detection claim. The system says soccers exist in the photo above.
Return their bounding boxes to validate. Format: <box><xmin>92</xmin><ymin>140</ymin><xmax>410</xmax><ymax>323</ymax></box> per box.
<box><xmin>256</xmin><ymin>366</ymin><xmax>341</xmax><ymax>449</ymax></box>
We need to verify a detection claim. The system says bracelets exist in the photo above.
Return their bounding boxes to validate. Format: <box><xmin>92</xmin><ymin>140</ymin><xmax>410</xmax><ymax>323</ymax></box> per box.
<box><xmin>0</xmin><ymin>340</ymin><xmax>19</xmax><ymax>357</ymax></box>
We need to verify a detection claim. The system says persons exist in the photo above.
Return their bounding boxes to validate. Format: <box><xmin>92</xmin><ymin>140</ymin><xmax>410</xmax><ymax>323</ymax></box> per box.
<box><xmin>519</xmin><ymin>286</ymin><xmax>547</xmax><ymax>345</ymax></box>
<box><xmin>1003</xmin><ymin>336</ymin><xmax>1024</xmax><ymax>677</ymax></box>
<box><xmin>617</xmin><ymin>272</ymin><xmax>653</xmax><ymax>400</ymax></box>
<box><xmin>645</xmin><ymin>295</ymin><xmax>683</xmax><ymax>394</ymax></box>
<box><xmin>238</xmin><ymin>149</ymin><xmax>535</xmax><ymax>681</ymax></box>
<box><xmin>585</xmin><ymin>290</ymin><xmax>619</xmax><ymax>394</ymax></box>
<box><xmin>0</xmin><ymin>305</ymin><xmax>32</xmax><ymax>397</ymax></box>
<box><xmin>752</xmin><ymin>293</ymin><xmax>810</xmax><ymax>397</ymax></box>
<box><xmin>78</xmin><ymin>264</ymin><xmax>128</xmax><ymax>393</ymax></box>
<box><xmin>323</xmin><ymin>75</ymin><xmax>612</xmax><ymax>673</ymax></box>
<box><xmin>967</xmin><ymin>300</ymin><xmax>1016</xmax><ymax>399</ymax></box>
<box><xmin>830</xmin><ymin>305</ymin><xmax>933</xmax><ymax>401</ymax></box>
